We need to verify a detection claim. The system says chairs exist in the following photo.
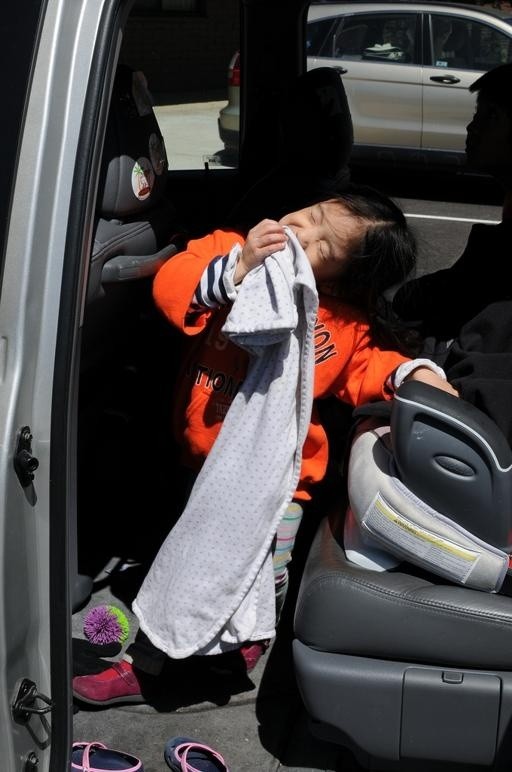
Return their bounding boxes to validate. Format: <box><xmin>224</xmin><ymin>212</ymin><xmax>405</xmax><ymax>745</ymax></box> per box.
<box><xmin>82</xmin><ymin>61</ymin><xmax>512</xmax><ymax>768</ymax></box>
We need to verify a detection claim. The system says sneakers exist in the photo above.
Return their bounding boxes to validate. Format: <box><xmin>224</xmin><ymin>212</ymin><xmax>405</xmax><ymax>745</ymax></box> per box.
<box><xmin>72</xmin><ymin>663</ymin><xmax>161</xmax><ymax>705</ymax></box>
<box><xmin>240</xmin><ymin>640</ymin><xmax>270</xmax><ymax>675</ymax></box>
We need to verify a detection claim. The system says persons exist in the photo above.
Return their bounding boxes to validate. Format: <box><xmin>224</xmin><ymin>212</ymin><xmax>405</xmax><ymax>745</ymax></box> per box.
<box><xmin>71</xmin><ymin>192</ymin><xmax>463</xmax><ymax>705</ymax></box>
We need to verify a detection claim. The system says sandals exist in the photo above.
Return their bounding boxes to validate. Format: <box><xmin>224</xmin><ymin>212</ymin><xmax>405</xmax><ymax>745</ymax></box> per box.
<box><xmin>165</xmin><ymin>737</ymin><xmax>229</xmax><ymax>772</ymax></box>
<box><xmin>71</xmin><ymin>742</ymin><xmax>143</xmax><ymax>772</ymax></box>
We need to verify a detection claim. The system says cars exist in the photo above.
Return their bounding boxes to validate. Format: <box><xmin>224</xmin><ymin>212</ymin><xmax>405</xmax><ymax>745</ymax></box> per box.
<box><xmin>218</xmin><ymin>3</ymin><xmax>512</xmax><ymax>160</ymax></box>
